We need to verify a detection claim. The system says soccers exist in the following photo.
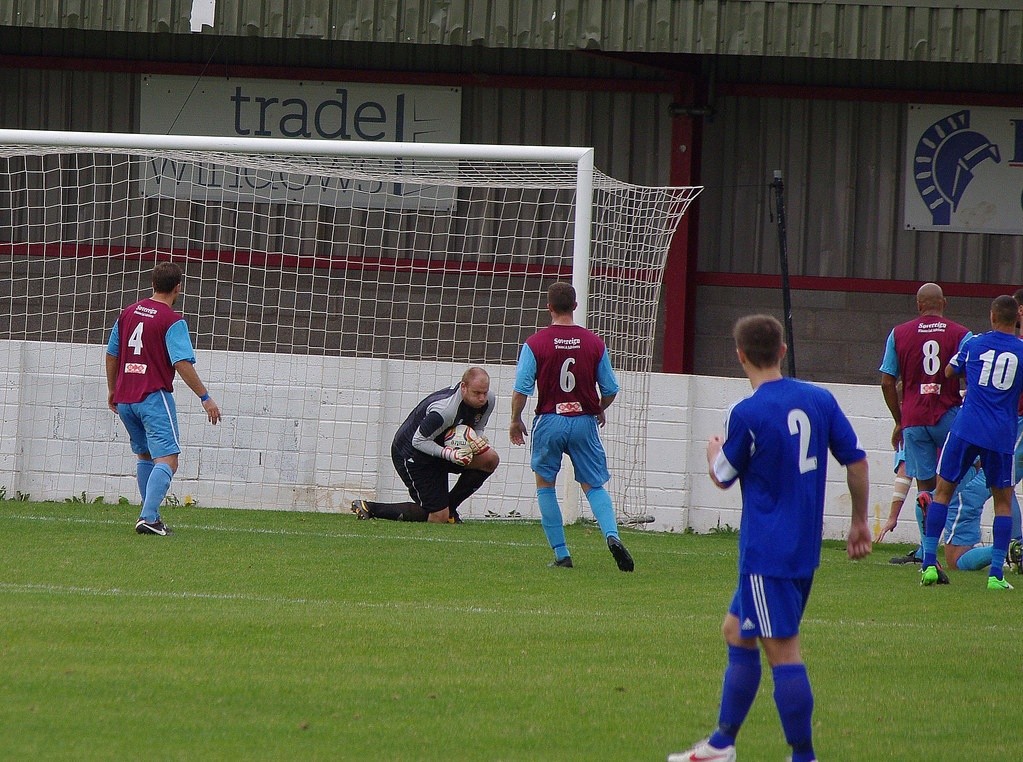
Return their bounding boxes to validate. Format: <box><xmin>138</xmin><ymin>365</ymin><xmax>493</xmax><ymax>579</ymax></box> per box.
<box><xmin>444</xmin><ymin>423</ymin><xmax>480</xmax><ymax>454</ymax></box>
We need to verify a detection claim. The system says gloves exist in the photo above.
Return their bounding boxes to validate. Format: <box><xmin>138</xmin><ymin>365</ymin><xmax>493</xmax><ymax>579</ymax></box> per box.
<box><xmin>442</xmin><ymin>447</ymin><xmax>472</xmax><ymax>466</ymax></box>
<box><xmin>470</xmin><ymin>436</ymin><xmax>491</xmax><ymax>455</ymax></box>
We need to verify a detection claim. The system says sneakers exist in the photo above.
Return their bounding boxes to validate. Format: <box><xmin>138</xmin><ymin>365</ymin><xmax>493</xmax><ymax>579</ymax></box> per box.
<box><xmin>1006</xmin><ymin>539</ymin><xmax>1023</xmax><ymax>575</ymax></box>
<box><xmin>134</xmin><ymin>516</ymin><xmax>175</xmax><ymax>537</ymax></box>
<box><xmin>668</xmin><ymin>738</ymin><xmax>736</xmax><ymax>762</ymax></box>
<box><xmin>918</xmin><ymin>566</ymin><xmax>938</xmax><ymax>586</ymax></box>
<box><xmin>607</xmin><ymin>536</ymin><xmax>634</xmax><ymax>572</ymax></box>
<box><xmin>547</xmin><ymin>556</ymin><xmax>573</xmax><ymax>568</ymax></box>
<box><xmin>936</xmin><ymin>560</ymin><xmax>949</xmax><ymax>585</ymax></box>
<box><xmin>447</xmin><ymin>510</ymin><xmax>465</xmax><ymax>524</ymax></box>
<box><xmin>986</xmin><ymin>575</ymin><xmax>1014</xmax><ymax>591</ymax></box>
<box><xmin>915</xmin><ymin>491</ymin><xmax>933</xmax><ymax>536</ymax></box>
<box><xmin>888</xmin><ymin>550</ymin><xmax>923</xmax><ymax>565</ymax></box>
<box><xmin>351</xmin><ymin>500</ymin><xmax>377</xmax><ymax>521</ymax></box>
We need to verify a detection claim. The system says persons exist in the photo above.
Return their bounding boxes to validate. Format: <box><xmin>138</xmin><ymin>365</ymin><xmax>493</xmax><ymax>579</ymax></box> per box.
<box><xmin>351</xmin><ymin>367</ymin><xmax>499</xmax><ymax>524</ymax></box>
<box><xmin>508</xmin><ymin>282</ymin><xmax>635</xmax><ymax>572</ymax></box>
<box><xmin>874</xmin><ymin>283</ymin><xmax>1023</xmax><ymax>589</ymax></box>
<box><xmin>106</xmin><ymin>263</ymin><xmax>222</xmax><ymax>536</ymax></box>
<box><xmin>668</xmin><ymin>316</ymin><xmax>872</xmax><ymax>762</ymax></box>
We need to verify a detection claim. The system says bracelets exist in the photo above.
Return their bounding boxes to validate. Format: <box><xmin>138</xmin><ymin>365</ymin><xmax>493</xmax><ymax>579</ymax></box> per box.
<box><xmin>891</xmin><ymin>478</ymin><xmax>912</xmax><ymax>503</ymax></box>
<box><xmin>200</xmin><ymin>390</ymin><xmax>210</xmax><ymax>403</ymax></box>
